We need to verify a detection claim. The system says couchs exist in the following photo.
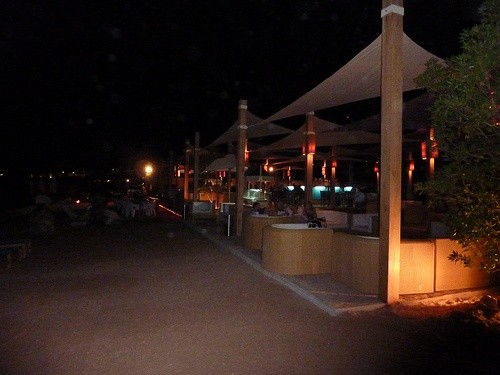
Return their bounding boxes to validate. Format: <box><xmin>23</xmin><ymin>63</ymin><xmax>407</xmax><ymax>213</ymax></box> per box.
<box><xmin>245</xmin><ymin>211</ymin><xmax>436</xmax><ymax>296</ymax></box>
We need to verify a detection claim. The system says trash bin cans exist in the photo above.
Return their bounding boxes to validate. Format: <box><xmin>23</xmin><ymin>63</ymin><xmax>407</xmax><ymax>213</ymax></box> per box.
<box><xmin>183</xmin><ymin>203</ymin><xmax>189</xmax><ymax>220</ymax></box>
<box><xmin>224</xmin><ymin>213</ymin><xmax>233</xmax><ymax>236</ymax></box>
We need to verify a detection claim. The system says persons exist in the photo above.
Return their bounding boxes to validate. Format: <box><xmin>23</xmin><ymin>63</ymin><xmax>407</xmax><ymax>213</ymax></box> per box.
<box><xmin>255</xmin><ymin>200</ymin><xmax>317</xmax><ymax>219</ymax></box>
